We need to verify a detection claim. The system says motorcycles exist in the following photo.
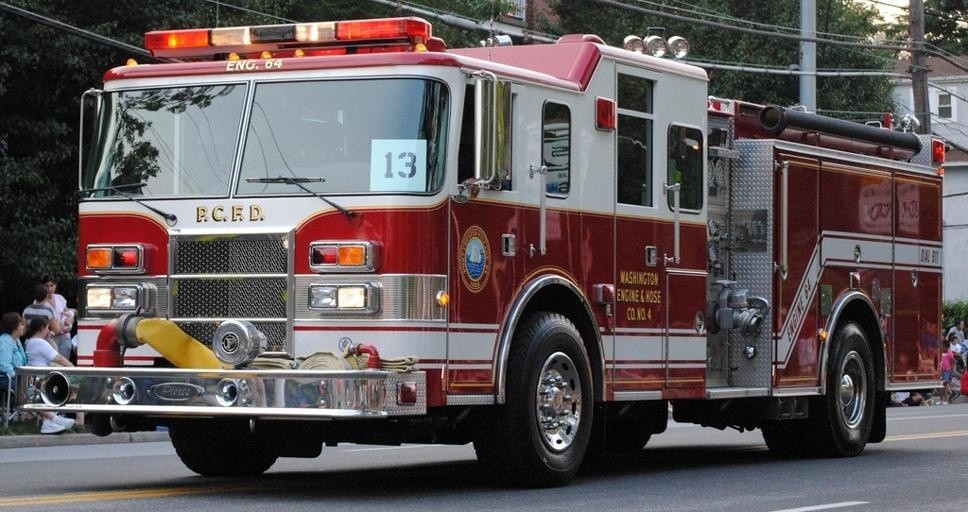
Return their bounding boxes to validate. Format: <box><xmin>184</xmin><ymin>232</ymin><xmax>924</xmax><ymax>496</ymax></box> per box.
<box><xmin>946</xmin><ymin>355</ymin><xmax>968</xmax><ymax>403</ymax></box>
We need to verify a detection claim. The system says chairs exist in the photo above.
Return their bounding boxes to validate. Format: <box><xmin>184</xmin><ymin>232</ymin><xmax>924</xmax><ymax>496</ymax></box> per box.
<box><xmin>0</xmin><ymin>370</ymin><xmax>40</xmax><ymax>430</ymax></box>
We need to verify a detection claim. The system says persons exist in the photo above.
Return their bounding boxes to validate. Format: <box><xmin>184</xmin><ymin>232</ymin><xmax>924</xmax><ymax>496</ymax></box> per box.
<box><xmin>0</xmin><ymin>274</ymin><xmax>78</xmax><ymax>434</ymax></box>
<box><xmin>891</xmin><ymin>318</ymin><xmax>968</xmax><ymax>407</ymax></box>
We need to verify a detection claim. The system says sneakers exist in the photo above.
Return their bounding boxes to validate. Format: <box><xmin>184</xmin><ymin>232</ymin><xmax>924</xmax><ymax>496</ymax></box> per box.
<box><xmin>40</xmin><ymin>420</ymin><xmax>67</xmax><ymax>434</ymax></box>
<box><xmin>921</xmin><ymin>398</ymin><xmax>950</xmax><ymax>407</ymax></box>
<box><xmin>51</xmin><ymin>414</ymin><xmax>76</xmax><ymax>431</ymax></box>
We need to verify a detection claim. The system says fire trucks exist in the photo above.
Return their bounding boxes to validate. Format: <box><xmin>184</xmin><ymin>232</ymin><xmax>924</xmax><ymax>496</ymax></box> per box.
<box><xmin>15</xmin><ymin>18</ymin><xmax>951</xmax><ymax>493</ymax></box>
